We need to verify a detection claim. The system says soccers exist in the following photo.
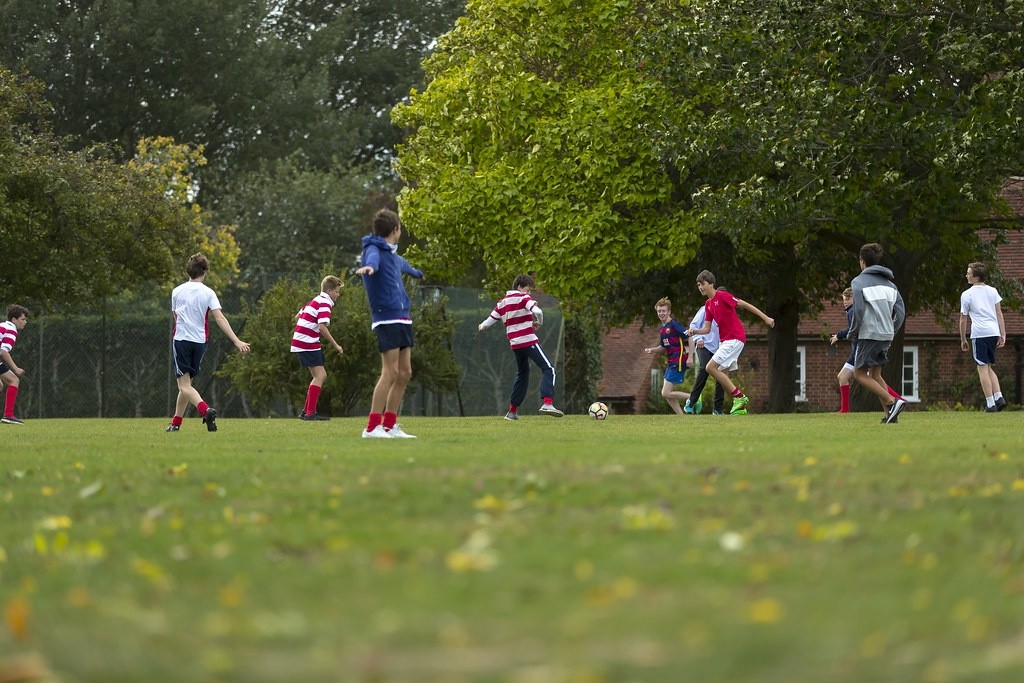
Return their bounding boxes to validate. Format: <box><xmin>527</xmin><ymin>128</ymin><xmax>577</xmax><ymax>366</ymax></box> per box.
<box><xmin>588</xmin><ymin>401</ymin><xmax>608</xmax><ymax>421</ymax></box>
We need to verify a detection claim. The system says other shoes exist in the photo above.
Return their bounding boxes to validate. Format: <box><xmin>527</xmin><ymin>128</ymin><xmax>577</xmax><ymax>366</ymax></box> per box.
<box><xmin>504</xmin><ymin>411</ymin><xmax>519</xmax><ymax>420</ymax></box>
<box><xmin>304</xmin><ymin>410</ymin><xmax>331</xmax><ymax>421</ymax></box>
<box><xmin>298</xmin><ymin>408</ymin><xmax>307</xmax><ymax>418</ymax></box>
<box><xmin>1</xmin><ymin>415</ymin><xmax>24</xmax><ymax>424</ymax></box>
<box><xmin>713</xmin><ymin>409</ymin><xmax>725</xmax><ymax>415</ymax></box>
<box><xmin>883</xmin><ymin>398</ymin><xmax>906</xmax><ymax>423</ymax></box>
<box><xmin>880</xmin><ymin>416</ymin><xmax>898</xmax><ymax>424</ymax></box>
<box><xmin>539</xmin><ymin>405</ymin><xmax>564</xmax><ymax>417</ymax></box>
<box><xmin>684</xmin><ymin>398</ymin><xmax>694</xmax><ymax>414</ymax></box>
<box><xmin>985</xmin><ymin>405</ymin><xmax>998</xmax><ymax>413</ymax></box>
<box><xmin>695</xmin><ymin>393</ymin><xmax>703</xmax><ymax>414</ymax></box>
<box><xmin>995</xmin><ymin>397</ymin><xmax>1007</xmax><ymax>412</ymax></box>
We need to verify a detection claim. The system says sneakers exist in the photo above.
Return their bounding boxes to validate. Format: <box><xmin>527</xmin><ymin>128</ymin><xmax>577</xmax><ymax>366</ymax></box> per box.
<box><xmin>729</xmin><ymin>394</ymin><xmax>749</xmax><ymax>414</ymax></box>
<box><xmin>362</xmin><ymin>424</ymin><xmax>395</xmax><ymax>438</ymax></box>
<box><xmin>386</xmin><ymin>423</ymin><xmax>417</xmax><ymax>438</ymax></box>
<box><xmin>166</xmin><ymin>423</ymin><xmax>179</xmax><ymax>432</ymax></box>
<box><xmin>202</xmin><ymin>407</ymin><xmax>218</xmax><ymax>432</ymax></box>
<box><xmin>730</xmin><ymin>408</ymin><xmax>748</xmax><ymax>415</ymax></box>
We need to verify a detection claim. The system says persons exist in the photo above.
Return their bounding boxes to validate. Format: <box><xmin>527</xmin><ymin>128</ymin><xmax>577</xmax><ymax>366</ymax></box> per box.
<box><xmin>846</xmin><ymin>244</ymin><xmax>906</xmax><ymax>424</ymax></box>
<box><xmin>959</xmin><ymin>262</ymin><xmax>1008</xmax><ymax>412</ymax></box>
<box><xmin>0</xmin><ymin>303</ymin><xmax>28</xmax><ymax>425</ymax></box>
<box><xmin>166</xmin><ymin>252</ymin><xmax>251</xmax><ymax>431</ymax></box>
<box><xmin>356</xmin><ymin>206</ymin><xmax>427</xmax><ymax>439</ymax></box>
<box><xmin>290</xmin><ymin>275</ymin><xmax>344</xmax><ymax>421</ymax></box>
<box><xmin>688</xmin><ymin>270</ymin><xmax>774</xmax><ymax>416</ymax></box>
<box><xmin>645</xmin><ymin>296</ymin><xmax>703</xmax><ymax>415</ymax></box>
<box><xmin>830</xmin><ymin>287</ymin><xmax>906</xmax><ymax>413</ymax></box>
<box><xmin>478</xmin><ymin>274</ymin><xmax>565</xmax><ymax>420</ymax></box>
<box><xmin>684</xmin><ymin>286</ymin><xmax>726</xmax><ymax>415</ymax></box>
